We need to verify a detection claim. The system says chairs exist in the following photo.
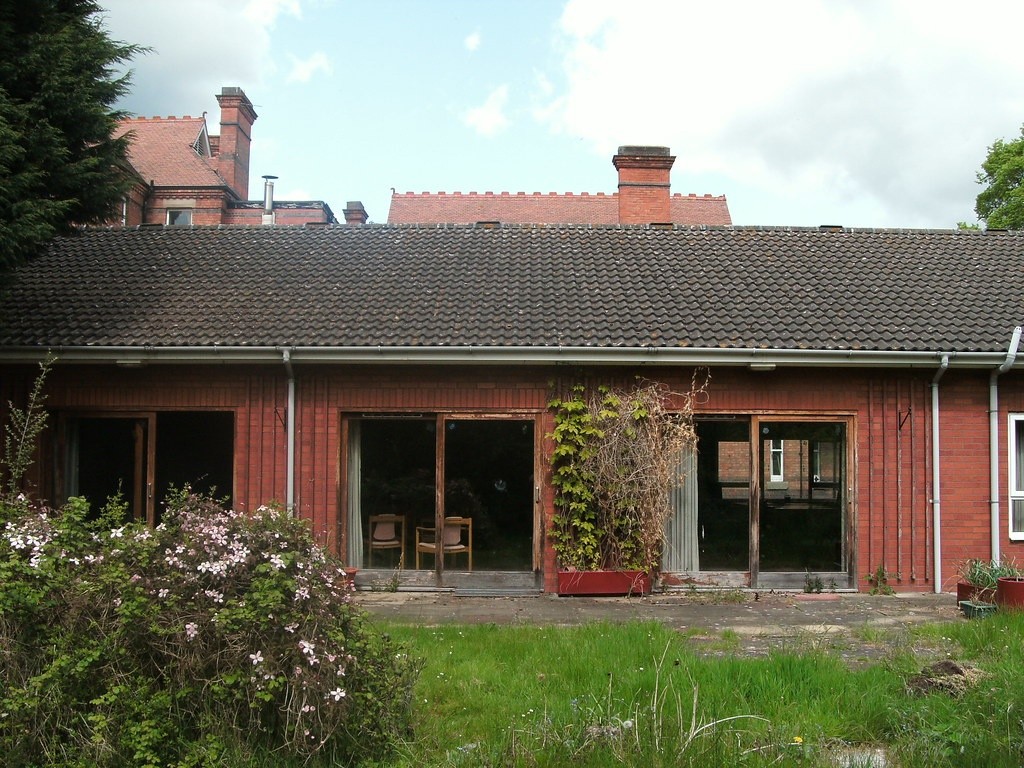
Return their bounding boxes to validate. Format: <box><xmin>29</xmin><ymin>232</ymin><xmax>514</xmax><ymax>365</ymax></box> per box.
<box><xmin>368</xmin><ymin>513</ymin><xmax>407</xmax><ymax>569</ymax></box>
<box><xmin>416</xmin><ymin>516</ymin><xmax>473</xmax><ymax>572</ymax></box>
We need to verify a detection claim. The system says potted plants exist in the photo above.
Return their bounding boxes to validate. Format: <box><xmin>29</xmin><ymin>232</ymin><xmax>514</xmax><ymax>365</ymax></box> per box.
<box><xmin>955</xmin><ymin>558</ymin><xmax>1024</xmax><ymax>604</ymax></box>
<box><xmin>544</xmin><ymin>367</ymin><xmax>709</xmax><ymax>595</ymax></box>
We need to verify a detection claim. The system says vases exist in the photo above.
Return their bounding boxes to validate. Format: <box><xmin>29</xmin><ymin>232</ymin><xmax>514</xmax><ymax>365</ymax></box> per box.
<box><xmin>958</xmin><ymin>601</ymin><xmax>996</xmax><ymax>618</ymax></box>
<box><xmin>996</xmin><ymin>576</ymin><xmax>1024</xmax><ymax>612</ymax></box>
